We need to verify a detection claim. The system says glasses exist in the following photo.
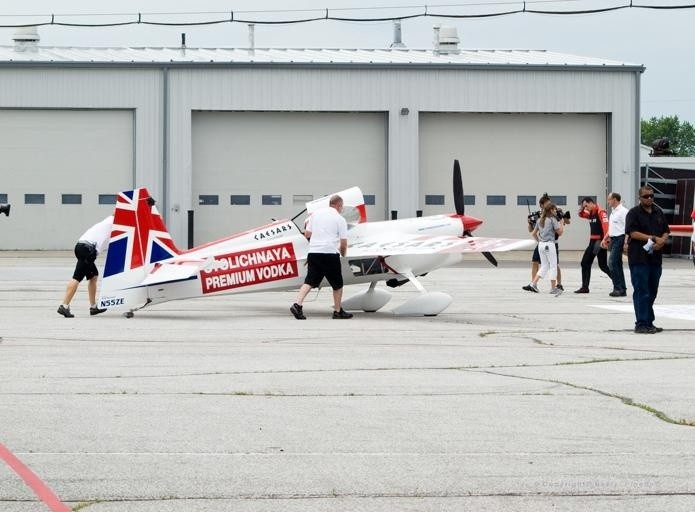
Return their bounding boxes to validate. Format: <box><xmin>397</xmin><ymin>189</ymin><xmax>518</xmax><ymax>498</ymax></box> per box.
<box><xmin>641</xmin><ymin>194</ymin><xmax>654</xmax><ymax>198</ymax></box>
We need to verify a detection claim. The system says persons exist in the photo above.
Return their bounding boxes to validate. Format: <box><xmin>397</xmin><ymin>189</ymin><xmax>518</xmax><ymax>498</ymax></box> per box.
<box><xmin>574</xmin><ymin>196</ymin><xmax>612</xmax><ymax>293</ymax></box>
<box><xmin>57</xmin><ymin>197</ymin><xmax>155</xmax><ymax>317</ymax></box>
<box><xmin>601</xmin><ymin>192</ymin><xmax>630</xmax><ymax>296</ymax></box>
<box><xmin>626</xmin><ymin>186</ymin><xmax>671</xmax><ymax>334</ymax></box>
<box><xmin>523</xmin><ymin>196</ymin><xmax>571</xmax><ymax>293</ymax></box>
<box><xmin>290</xmin><ymin>195</ymin><xmax>352</xmax><ymax>319</ymax></box>
<box><xmin>529</xmin><ymin>203</ymin><xmax>564</xmax><ymax>295</ymax></box>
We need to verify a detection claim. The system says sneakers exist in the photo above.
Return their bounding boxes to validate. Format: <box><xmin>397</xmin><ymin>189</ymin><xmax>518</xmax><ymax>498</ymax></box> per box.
<box><xmin>90</xmin><ymin>305</ymin><xmax>106</xmax><ymax>315</ymax></box>
<box><xmin>635</xmin><ymin>325</ymin><xmax>662</xmax><ymax>333</ymax></box>
<box><xmin>575</xmin><ymin>288</ymin><xmax>589</xmax><ymax>293</ymax></box>
<box><xmin>332</xmin><ymin>307</ymin><xmax>352</xmax><ymax>319</ymax></box>
<box><xmin>523</xmin><ymin>282</ymin><xmax>539</xmax><ymax>292</ymax></box>
<box><xmin>609</xmin><ymin>288</ymin><xmax>626</xmax><ymax>296</ymax></box>
<box><xmin>289</xmin><ymin>303</ymin><xmax>305</xmax><ymax>319</ymax></box>
<box><xmin>57</xmin><ymin>305</ymin><xmax>74</xmax><ymax>317</ymax></box>
<box><xmin>550</xmin><ymin>284</ymin><xmax>563</xmax><ymax>293</ymax></box>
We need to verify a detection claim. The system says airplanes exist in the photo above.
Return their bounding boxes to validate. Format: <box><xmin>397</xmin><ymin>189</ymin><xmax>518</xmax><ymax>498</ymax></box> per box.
<box><xmin>97</xmin><ymin>157</ymin><xmax>539</xmax><ymax>322</ymax></box>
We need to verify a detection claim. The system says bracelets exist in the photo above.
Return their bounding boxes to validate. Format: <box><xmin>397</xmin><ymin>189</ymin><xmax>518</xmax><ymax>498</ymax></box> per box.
<box><xmin>653</xmin><ymin>235</ymin><xmax>656</xmax><ymax>243</ymax></box>
